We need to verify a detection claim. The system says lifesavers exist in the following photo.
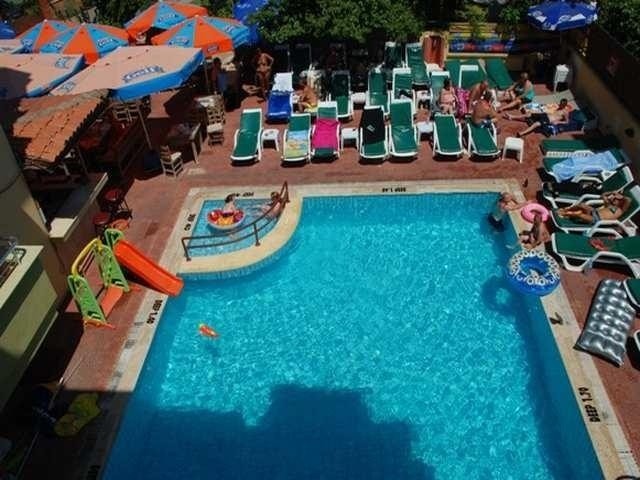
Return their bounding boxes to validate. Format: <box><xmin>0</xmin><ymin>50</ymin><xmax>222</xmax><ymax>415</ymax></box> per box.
<box><xmin>521</xmin><ymin>204</ymin><xmax>548</xmax><ymax>222</ymax></box>
<box><xmin>208</xmin><ymin>209</ymin><xmax>244</xmax><ymax>229</ymax></box>
<box><xmin>198</xmin><ymin>323</ymin><xmax>218</xmax><ymax>337</ymax></box>
<box><xmin>506</xmin><ymin>249</ymin><xmax>560</xmax><ymax>296</ymax></box>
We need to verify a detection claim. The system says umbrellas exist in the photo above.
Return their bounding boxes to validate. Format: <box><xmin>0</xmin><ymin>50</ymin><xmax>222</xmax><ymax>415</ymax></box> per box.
<box><xmin>0</xmin><ymin>0</ymin><xmax>251</xmax><ymax>151</ymax></box>
<box><xmin>234</xmin><ymin>0</ymin><xmax>272</xmax><ymax>44</ymax></box>
<box><xmin>528</xmin><ymin>0</ymin><xmax>598</xmax><ymax>50</ymax></box>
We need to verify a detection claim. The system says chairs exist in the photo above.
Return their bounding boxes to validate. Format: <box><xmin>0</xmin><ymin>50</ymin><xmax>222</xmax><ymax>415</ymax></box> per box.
<box><xmin>158</xmin><ymin>144</ymin><xmax>185</xmax><ymax>178</ymax></box>
<box><xmin>207</xmin><ymin>91</ymin><xmax>226</xmax><ymax>144</ymax></box>
<box><xmin>228</xmin><ymin>42</ymin><xmax>514</xmax><ymax>163</ymax></box>
<box><xmin>530</xmin><ymin>87</ymin><xmax>638</xmax><ymax>366</ymax></box>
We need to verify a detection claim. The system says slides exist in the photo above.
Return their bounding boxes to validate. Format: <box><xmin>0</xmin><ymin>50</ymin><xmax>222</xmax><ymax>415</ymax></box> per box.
<box><xmin>113</xmin><ymin>240</ymin><xmax>184</xmax><ymax>297</ymax></box>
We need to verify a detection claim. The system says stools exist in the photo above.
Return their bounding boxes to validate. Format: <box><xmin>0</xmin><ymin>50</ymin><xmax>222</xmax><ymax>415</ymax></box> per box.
<box><xmin>502</xmin><ymin>135</ymin><xmax>523</xmax><ymax>162</ymax></box>
<box><xmin>91</xmin><ymin>187</ymin><xmax>133</xmax><ymax>237</ymax></box>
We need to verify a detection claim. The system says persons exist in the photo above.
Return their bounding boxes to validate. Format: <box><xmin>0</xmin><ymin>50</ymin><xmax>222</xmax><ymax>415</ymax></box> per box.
<box><xmin>505</xmin><ymin>214</ymin><xmax>551</xmax><ymax>251</ymax></box>
<box><xmin>253</xmin><ymin>47</ymin><xmax>275</xmax><ymax>103</ymax></box>
<box><xmin>415</xmin><ymin>72</ymin><xmax>570</xmax><ymax>137</ymax></box>
<box><xmin>297</xmin><ymin>79</ymin><xmax>316</xmax><ymax>113</ymax></box>
<box><xmin>487</xmin><ymin>192</ymin><xmax>522</xmax><ymax>235</ymax></box>
<box><xmin>557</xmin><ymin>191</ymin><xmax>632</xmax><ymax>225</ymax></box>
<box><xmin>220</xmin><ymin>190</ymin><xmax>284</xmax><ymax>219</ymax></box>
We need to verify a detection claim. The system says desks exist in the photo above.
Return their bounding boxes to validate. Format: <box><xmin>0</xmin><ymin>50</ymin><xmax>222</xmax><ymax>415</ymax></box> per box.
<box><xmin>166</xmin><ymin>122</ymin><xmax>205</xmax><ymax>166</ymax></box>
